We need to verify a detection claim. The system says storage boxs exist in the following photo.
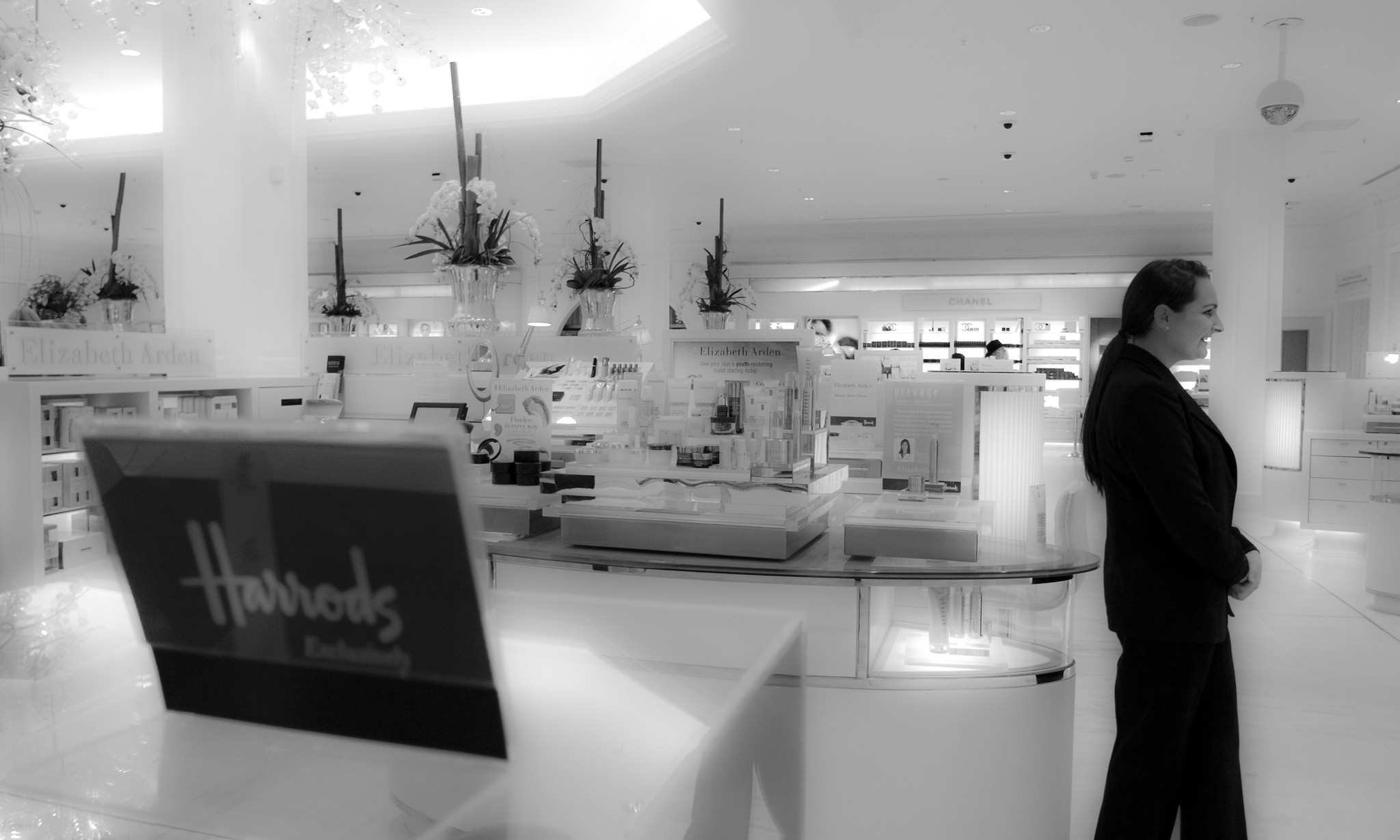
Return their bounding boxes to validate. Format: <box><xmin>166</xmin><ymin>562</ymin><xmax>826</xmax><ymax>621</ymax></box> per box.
<box><xmin>50</xmin><ymin>529</ymin><xmax>108</xmax><ymax>570</ymax></box>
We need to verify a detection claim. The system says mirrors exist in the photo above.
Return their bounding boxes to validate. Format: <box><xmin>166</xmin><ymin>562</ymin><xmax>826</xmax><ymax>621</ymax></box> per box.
<box><xmin>467</xmin><ymin>338</ymin><xmax>499</xmax><ymax>420</ymax></box>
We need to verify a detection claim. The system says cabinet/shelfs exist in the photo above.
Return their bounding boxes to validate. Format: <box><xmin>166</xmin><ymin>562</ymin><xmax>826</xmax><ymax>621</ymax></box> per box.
<box><xmin>1023</xmin><ymin>317</ymin><xmax>1085</xmax><ymax>445</ymax></box>
<box><xmin>1298</xmin><ymin>430</ymin><xmax>1400</xmax><ymax>533</ymax></box>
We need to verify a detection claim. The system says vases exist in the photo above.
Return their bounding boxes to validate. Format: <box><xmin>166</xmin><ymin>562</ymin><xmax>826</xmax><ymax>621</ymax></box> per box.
<box><xmin>96</xmin><ymin>298</ymin><xmax>134</xmax><ymax>333</ymax></box>
<box><xmin>326</xmin><ymin>316</ymin><xmax>353</xmax><ymax>337</ymax></box>
<box><xmin>436</xmin><ymin>264</ymin><xmax>505</xmax><ymax>336</ymax></box>
<box><xmin>574</xmin><ymin>288</ymin><xmax>619</xmax><ymax>336</ymax></box>
<box><xmin>700</xmin><ymin>311</ymin><xmax>731</xmax><ymax>329</ymax></box>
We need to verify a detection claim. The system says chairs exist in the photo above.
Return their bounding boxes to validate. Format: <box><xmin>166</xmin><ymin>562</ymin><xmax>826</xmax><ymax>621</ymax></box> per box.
<box><xmin>983</xmin><ymin>480</ymin><xmax>1091</xmax><ymax>637</ymax></box>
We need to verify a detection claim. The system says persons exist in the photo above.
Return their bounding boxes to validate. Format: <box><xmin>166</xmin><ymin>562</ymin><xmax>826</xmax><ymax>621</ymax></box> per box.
<box><xmin>881</xmin><ymin>339</ymin><xmax>1013</xmax><ymax>381</ymax></box>
<box><xmin>803</xmin><ymin>316</ymin><xmax>858</xmax><ymax>360</ymax></box>
<box><xmin>420</xmin><ymin>324</ymin><xmax>431</xmax><ymax>337</ymax></box>
<box><xmin>1080</xmin><ymin>259</ymin><xmax>1261</xmax><ymax>840</ymax></box>
<box><xmin>894</xmin><ymin>439</ymin><xmax>913</xmax><ymax>464</ymax></box>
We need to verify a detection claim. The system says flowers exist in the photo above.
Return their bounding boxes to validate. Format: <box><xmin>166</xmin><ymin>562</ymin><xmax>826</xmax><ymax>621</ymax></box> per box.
<box><xmin>308</xmin><ymin>207</ymin><xmax>381</xmax><ymax>329</ymax></box>
<box><xmin>388</xmin><ymin>61</ymin><xmax>544</xmax><ymax>283</ymax></box>
<box><xmin>675</xmin><ymin>198</ymin><xmax>755</xmax><ymax>325</ymax></box>
<box><xmin>67</xmin><ymin>170</ymin><xmax>160</xmax><ymax>318</ymax></box>
<box><xmin>547</xmin><ymin>138</ymin><xmax>640</xmax><ymax>312</ymax></box>
<box><xmin>19</xmin><ymin>259</ymin><xmax>98</xmax><ymax>328</ymax></box>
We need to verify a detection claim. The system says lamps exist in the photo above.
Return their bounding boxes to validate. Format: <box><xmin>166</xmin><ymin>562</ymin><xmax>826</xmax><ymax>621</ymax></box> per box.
<box><xmin>519</xmin><ymin>291</ymin><xmax>552</xmax><ymax>356</ymax></box>
<box><xmin>610</xmin><ymin>315</ymin><xmax>653</xmax><ymax>362</ymax></box>
<box><xmin>1388</xmin><ymin>344</ymin><xmax>1399</xmax><ymax>364</ymax></box>
